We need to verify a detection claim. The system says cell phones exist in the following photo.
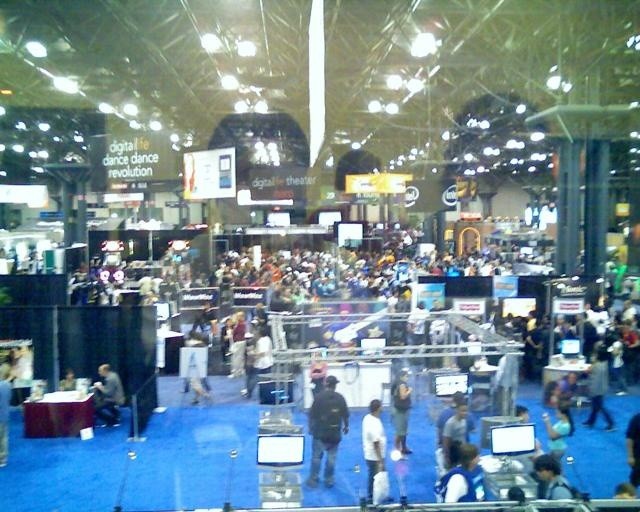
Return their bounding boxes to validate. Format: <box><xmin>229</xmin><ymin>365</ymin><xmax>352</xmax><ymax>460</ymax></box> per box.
<box><xmin>219</xmin><ymin>155</ymin><xmax>231</xmax><ymax>188</ymax></box>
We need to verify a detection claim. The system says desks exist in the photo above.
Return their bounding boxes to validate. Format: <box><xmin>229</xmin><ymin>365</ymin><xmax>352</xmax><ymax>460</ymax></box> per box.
<box><xmin>23</xmin><ymin>391</ymin><xmax>94</xmax><ymax>438</ymax></box>
<box><xmin>542</xmin><ymin>360</ymin><xmax>592</xmax><ymax>408</ymax></box>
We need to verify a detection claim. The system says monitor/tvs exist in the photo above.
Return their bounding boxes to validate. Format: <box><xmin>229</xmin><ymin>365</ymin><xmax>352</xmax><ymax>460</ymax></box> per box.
<box><xmin>490</xmin><ymin>423</ymin><xmax>536</xmax><ymax>455</ymax></box>
<box><xmin>361</xmin><ymin>338</ymin><xmax>386</xmax><ymax>363</ymax></box>
<box><xmin>435</xmin><ymin>373</ymin><xmax>469</xmax><ymax>397</ymax></box>
<box><xmin>560</xmin><ymin>339</ymin><xmax>581</xmax><ymax>358</ymax></box>
<box><xmin>499</xmin><ymin>297</ymin><xmax>537</xmax><ymax>319</ymax></box>
<box><xmin>257</xmin><ymin>434</ymin><xmax>305</xmax><ymax>466</ymax></box>
<box><xmin>257</xmin><ymin>380</ymin><xmax>294</xmax><ymax>405</ymax></box>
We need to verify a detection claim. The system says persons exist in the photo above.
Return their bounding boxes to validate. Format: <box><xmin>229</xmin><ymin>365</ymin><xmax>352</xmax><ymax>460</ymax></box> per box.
<box><xmin>247</xmin><ymin>244</ymin><xmax>639</xmax><ymax>512</ymax></box>
<box><xmin>1</xmin><ymin>245</ymin><xmax>250</xmax><ymax>427</ymax></box>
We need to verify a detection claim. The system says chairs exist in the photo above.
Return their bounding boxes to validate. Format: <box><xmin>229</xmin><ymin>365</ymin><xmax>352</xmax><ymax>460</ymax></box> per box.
<box><xmin>77</xmin><ymin>378</ymin><xmax>90</xmax><ymax>392</ymax></box>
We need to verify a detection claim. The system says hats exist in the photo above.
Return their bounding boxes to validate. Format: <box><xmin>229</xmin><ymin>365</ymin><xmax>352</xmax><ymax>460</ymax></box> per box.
<box><xmin>326</xmin><ymin>376</ymin><xmax>340</xmax><ymax>384</ymax></box>
<box><xmin>399</xmin><ymin>367</ymin><xmax>412</xmax><ymax>377</ymax></box>
